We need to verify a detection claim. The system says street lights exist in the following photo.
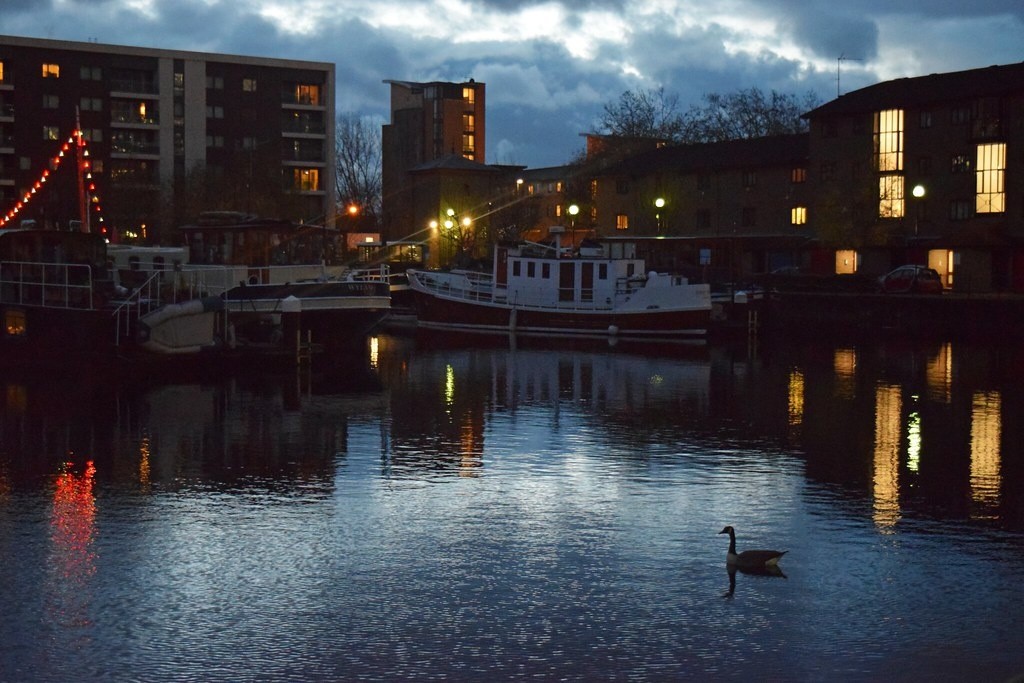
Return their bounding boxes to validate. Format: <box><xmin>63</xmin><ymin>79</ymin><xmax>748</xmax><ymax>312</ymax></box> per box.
<box><xmin>911</xmin><ymin>183</ymin><xmax>927</xmax><ymax>298</ymax></box>
<box><xmin>654</xmin><ymin>197</ymin><xmax>666</xmax><ymax>236</ymax></box>
<box><xmin>568</xmin><ymin>203</ymin><xmax>580</xmax><ymax>250</ymax></box>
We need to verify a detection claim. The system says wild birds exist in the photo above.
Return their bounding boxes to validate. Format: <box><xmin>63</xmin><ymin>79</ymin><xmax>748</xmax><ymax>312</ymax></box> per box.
<box><xmin>718</xmin><ymin>526</ymin><xmax>789</xmax><ymax>566</ymax></box>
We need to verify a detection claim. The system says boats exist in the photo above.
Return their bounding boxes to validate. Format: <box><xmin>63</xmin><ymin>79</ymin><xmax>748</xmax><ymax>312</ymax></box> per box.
<box><xmin>404</xmin><ymin>226</ymin><xmax>715</xmax><ymax>359</ymax></box>
<box><xmin>0</xmin><ymin>210</ymin><xmax>394</xmax><ymax>375</ymax></box>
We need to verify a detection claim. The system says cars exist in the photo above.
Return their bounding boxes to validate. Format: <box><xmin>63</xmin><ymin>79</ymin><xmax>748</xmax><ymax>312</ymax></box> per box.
<box><xmin>875</xmin><ymin>263</ymin><xmax>943</xmax><ymax>295</ymax></box>
